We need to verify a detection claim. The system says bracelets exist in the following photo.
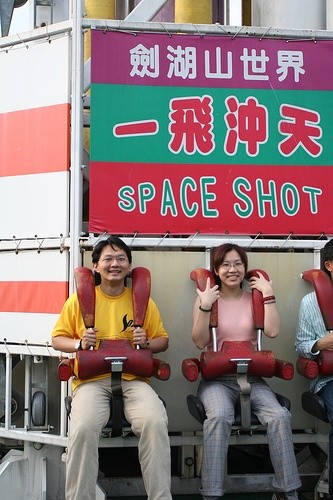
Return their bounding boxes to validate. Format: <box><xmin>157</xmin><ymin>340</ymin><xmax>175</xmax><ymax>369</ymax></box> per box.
<box><xmin>146</xmin><ymin>338</ymin><xmax>150</xmax><ymax>348</ymax></box>
<box><xmin>263</xmin><ymin>300</ymin><xmax>276</xmax><ymax>305</ymax></box>
<box><xmin>198</xmin><ymin>306</ymin><xmax>212</xmax><ymax>312</ymax></box>
<box><xmin>263</xmin><ymin>295</ymin><xmax>275</xmax><ymax>302</ymax></box>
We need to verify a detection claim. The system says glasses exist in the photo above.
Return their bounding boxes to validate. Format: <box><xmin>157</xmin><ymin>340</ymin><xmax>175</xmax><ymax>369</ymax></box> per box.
<box><xmin>222</xmin><ymin>262</ymin><xmax>245</xmax><ymax>268</ymax></box>
<box><xmin>99</xmin><ymin>257</ymin><xmax>128</xmax><ymax>263</ymax></box>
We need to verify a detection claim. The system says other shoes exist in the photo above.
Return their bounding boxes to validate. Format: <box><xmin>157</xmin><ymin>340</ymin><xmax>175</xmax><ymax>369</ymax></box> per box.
<box><xmin>314</xmin><ymin>485</ymin><xmax>331</xmax><ymax>500</ymax></box>
<box><xmin>271</xmin><ymin>491</ymin><xmax>300</xmax><ymax>500</ymax></box>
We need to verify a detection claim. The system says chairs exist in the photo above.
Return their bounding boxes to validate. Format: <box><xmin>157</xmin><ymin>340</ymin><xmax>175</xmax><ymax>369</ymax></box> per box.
<box><xmin>181</xmin><ymin>356</ymin><xmax>294</xmax><ymax>430</ymax></box>
<box><xmin>296</xmin><ymin>350</ymin><xmax>333</xmax><ymax>425</ymax></box>
<box><xmin>57</xmin><ymin>358</ymin><xmax>171</xmax><ymax>439</ymax></box>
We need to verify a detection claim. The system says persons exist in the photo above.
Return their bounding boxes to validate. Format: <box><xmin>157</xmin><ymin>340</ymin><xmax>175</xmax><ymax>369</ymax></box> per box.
<box><xmin>51</xmin><ymin>235</ymin><xmax>173</xmax><ymax>500</ymax></box>
<box><xmin>294</xmin><ymin>238</ymin><xmax>333</xmax><ymax>500</ymax></box>
<box><xmin>191</xmin><ymin>243</ymin><xmax>303</xmax><ymax>500</ymax></box>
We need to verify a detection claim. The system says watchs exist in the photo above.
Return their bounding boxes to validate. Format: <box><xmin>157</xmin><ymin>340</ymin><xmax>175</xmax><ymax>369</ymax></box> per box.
<box><xmin>74</xmin><ymin>338</ymin><xmax>83</xmax><ymax>350</ymax></box>
